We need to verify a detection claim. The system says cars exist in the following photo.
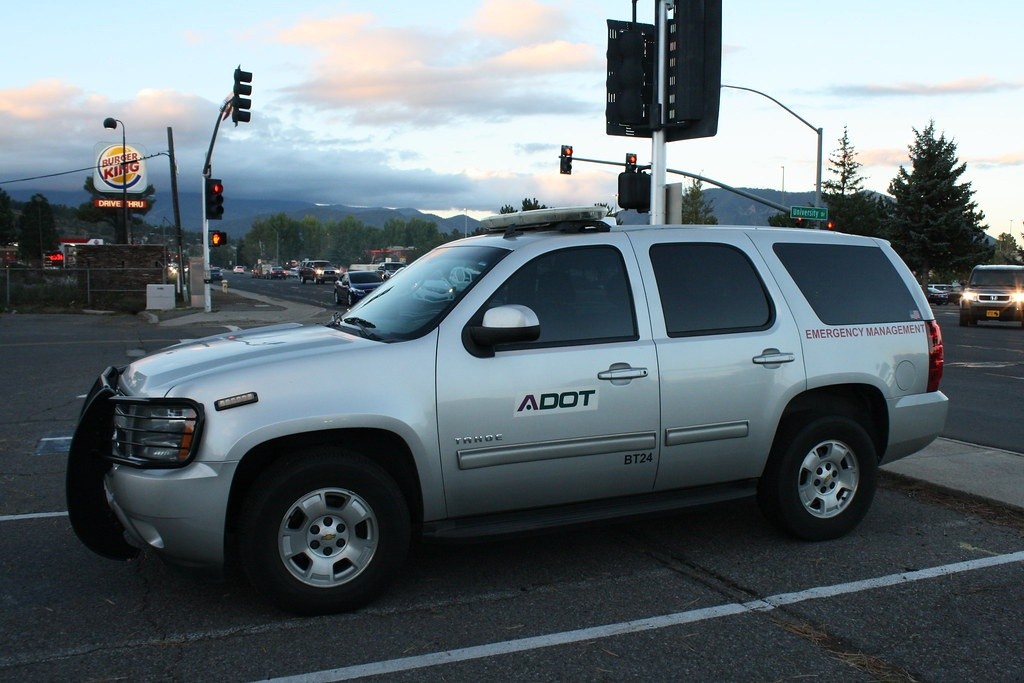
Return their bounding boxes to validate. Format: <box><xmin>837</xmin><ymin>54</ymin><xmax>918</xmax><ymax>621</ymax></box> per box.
<box><xmin>251</xmin><ymin>265</ymin><xmax>299</xmax><ymax>280</ymax></box>
<box><xmin>926</xmin><ymin>283</ymin><xmax>961</xmax><ymax>306</ymax></box>
<box><xmin>334</xmin><ymin>271</ymin><xmax>385</xmax><ymax>308</ymax></box>
<box><xmin>376</xmin><ymin>262</ymin><xmax>407</xmax><ymax>280</ymax></box>
<box><xmin>233</xmin><ymin>266</ymin><xmax>245</xmax><ymax>274</ymax></box>
<box><xmin>211</xmin><ymin>267</ymin><xmax>222</xmax><ymax>281</ymax></box>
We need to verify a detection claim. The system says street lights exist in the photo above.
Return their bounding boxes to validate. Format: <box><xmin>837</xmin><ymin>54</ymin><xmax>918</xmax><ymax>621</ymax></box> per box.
<box><xmin>781</xmin><ymin>165</ymin><xmax>785</xmax><ymax>206</ymax></box>
<box><xmin>103</xmin><ymin>118</ymin><xmax>129</xmax><ymax>243</ymax></box>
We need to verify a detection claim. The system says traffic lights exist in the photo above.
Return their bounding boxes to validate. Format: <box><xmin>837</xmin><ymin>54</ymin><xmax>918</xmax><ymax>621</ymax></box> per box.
<box><xmin>625</xmin><ymin>153</ymin><xmax>636</xmax><ymax>173</ymax></box>
<box><xmin>561</xmin><ymin>145</ymin><xmax>573</xmax><ymax>175</ymax></box>
<box><xmin>206</xmin><ymin>178</ymin><xmax>224</xmax><ymax>220</ymax></box>
<box><xmin>212</xmin><ymin>231</ymin><xmax>228</xmax><ymax>246</ymax></box>
<box><xmin>795</xmin><ymin>218</ymin><xmax>805</xmax><ymax>227</ymax></box>
<box><xmin>820</xmin><ymin>221</ymin><xmax>834</xmax><ymax>231</ymax></box>
<box><xmin>233</xmin><ymin>64</ymin><xmax>253</xmax><ymax>125</ymax></box>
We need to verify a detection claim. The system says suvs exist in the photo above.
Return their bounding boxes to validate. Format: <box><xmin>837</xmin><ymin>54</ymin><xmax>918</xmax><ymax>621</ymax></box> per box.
<box><xmin>959</xmin><ymin>264</ymin><xmax>1024</xmax><ymax>327</ymax></box>
<box><xmin>67</xmin><ymin>206</ymin><xmax>951</xmax><ymax>614</ymax></box>
<box><xmin>299</xmin><ymin>260</ymin><xmax>342</xmax><ymax>285</ymax></box>
